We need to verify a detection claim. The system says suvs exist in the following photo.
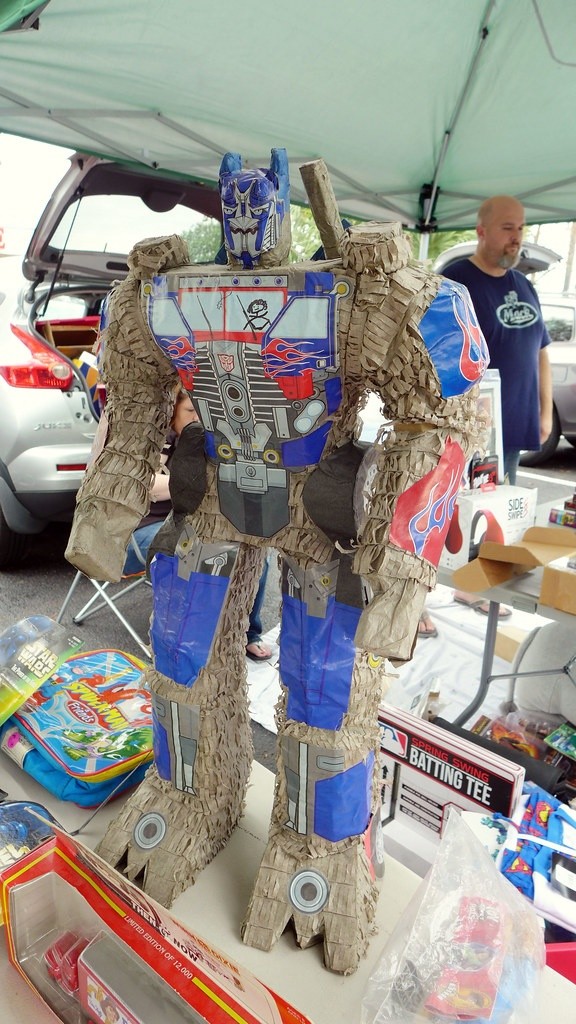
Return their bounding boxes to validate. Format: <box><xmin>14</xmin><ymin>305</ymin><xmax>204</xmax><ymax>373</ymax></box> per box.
<box><xmin>432</xmin><ymin>240</ymin><xmax>576</xmax><ymax>467</ymax></box>
<box><xmin>0</xmin><ymin>151</ymin><xmax>224</xmax><ymax>574</ymax></box>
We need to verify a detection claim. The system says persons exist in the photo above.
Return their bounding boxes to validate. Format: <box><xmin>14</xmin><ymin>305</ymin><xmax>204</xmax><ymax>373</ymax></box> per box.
<box><xmin>59</xmin><ymin>150</ymin><xmax>494</xmax><ymax>978</ymax></box>
<box><xmin>437</xmin><ymin>195</ymin><xmax>552</xmax><ymax>485</ymax></box>
